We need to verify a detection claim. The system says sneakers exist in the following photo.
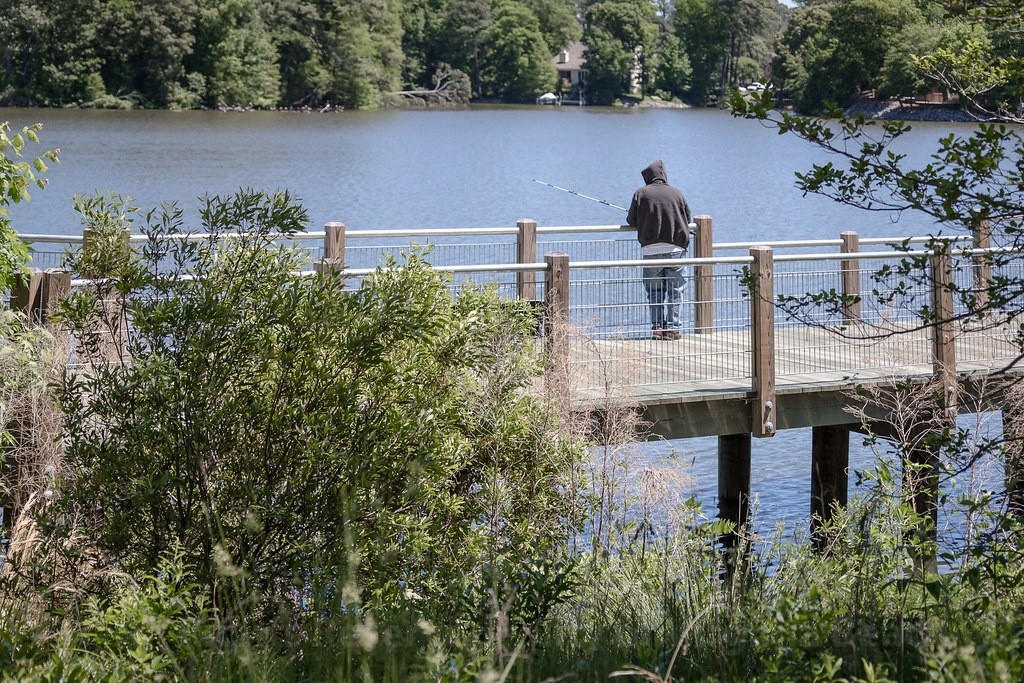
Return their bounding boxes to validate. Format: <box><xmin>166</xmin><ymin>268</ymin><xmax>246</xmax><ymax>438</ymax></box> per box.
<box><xmin>663</xmin><ymin>331</ymin><xmax>681</xmax><ymax>340</ymax></box>
<box><xmin>652</xmin><ymin>328</ymin><xmax>662</xmax><ymax>340</ymax></box>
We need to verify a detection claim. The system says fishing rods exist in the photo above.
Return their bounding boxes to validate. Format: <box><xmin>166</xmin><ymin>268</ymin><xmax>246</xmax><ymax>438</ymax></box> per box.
<box><xmin>530</xmin><ymin>177</ymin><xmax>698</xmax><ymax>236</ymax></box>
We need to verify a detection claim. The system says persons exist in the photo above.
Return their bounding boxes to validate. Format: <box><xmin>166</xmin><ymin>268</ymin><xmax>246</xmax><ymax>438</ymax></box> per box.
<box><xmin>626</xmin><ymin>160</ymin><xmax>691</xmax><ymax>340</ymax></box>
